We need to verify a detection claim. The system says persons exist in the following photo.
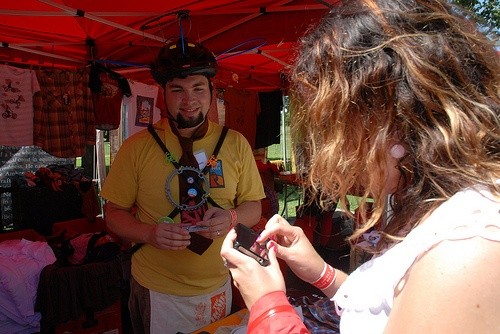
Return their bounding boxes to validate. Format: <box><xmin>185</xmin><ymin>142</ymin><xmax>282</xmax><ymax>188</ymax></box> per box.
<box><xmin>98</xmin><ymin>36</ymin><xmax>267</xmax><ymax>334</ymax></box>
<box><xmin>217</xmin><ymin>0</ymin><xmax>500</xmax><ymax>334</ymax></box>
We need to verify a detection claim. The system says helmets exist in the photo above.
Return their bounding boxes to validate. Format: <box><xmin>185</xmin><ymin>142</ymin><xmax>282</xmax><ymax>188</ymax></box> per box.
<box><xmin>151</xmin><ymin>41</ymin><xmax>218</xmax><ymax>84</ymax></box>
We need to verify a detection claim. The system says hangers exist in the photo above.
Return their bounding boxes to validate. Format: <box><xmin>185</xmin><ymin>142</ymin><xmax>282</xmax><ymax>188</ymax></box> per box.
<box><xmin>85</xmin><ymin>47</ymin><xmax>98</xmax><ymax>68</ymax></box>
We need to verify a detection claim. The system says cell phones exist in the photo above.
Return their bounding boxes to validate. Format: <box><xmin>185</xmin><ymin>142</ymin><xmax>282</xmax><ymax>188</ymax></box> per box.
<box><xmin>232</xmin><ymin>223</ymin><xmax>271</xmax><ymax>266</ymax></box>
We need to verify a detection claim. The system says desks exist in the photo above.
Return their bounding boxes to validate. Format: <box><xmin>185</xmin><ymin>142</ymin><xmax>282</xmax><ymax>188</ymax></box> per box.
<box><xmin>10</xmin><ymin>180</ymin><xmax>83</xmax><ymax>232</ymax></box>
<box><xmin>278</xmin><ymin>175</ymin><xmax>372</xmax><ymax>217</ymax></box>
<box><xmin>0</xmin><ymin>216</ymin><xmax>118</xmax><ymax>334</ymax></box>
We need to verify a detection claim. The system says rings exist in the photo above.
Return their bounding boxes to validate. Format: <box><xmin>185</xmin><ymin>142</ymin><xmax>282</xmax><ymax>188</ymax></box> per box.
<box><xmin>217</xmin><ymin>231</ymin><xmax>220</xmax><ymax>236</ymax></box>
<box><xmin>224</xmin><ymin>263</ymin><xmax>231</xmax><ymax>267</ymax></box>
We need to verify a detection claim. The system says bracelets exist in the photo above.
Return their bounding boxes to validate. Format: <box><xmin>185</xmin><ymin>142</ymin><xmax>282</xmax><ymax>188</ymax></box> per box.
<box><xmin>311</xmin><ymin>263</ymin><xmax>337</xmax><ymax>290</ymax></box>
<box><xmin>228</xmin><ymin>209</ymin><xmax>238</xmax><ymax>228</ymax></box>
